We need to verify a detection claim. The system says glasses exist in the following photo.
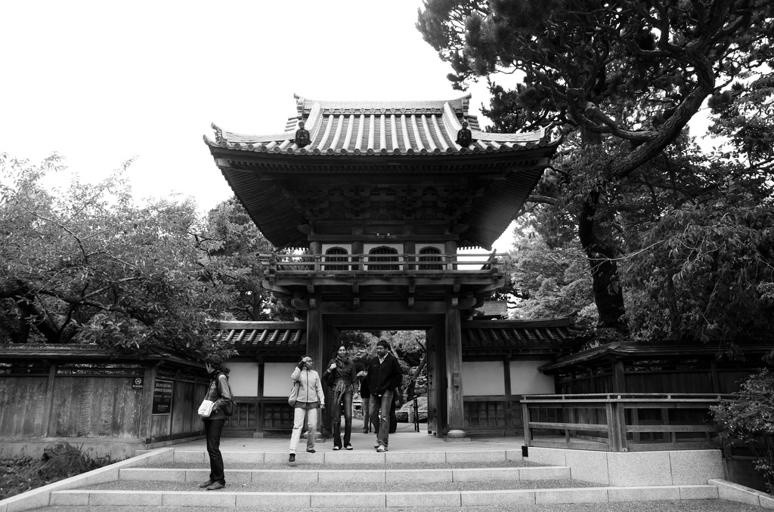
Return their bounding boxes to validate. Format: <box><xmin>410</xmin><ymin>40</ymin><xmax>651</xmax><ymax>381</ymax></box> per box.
<box><xmin>376</xmin><ymin>347</ymin><xmax>386</xmax><ymax>350</ymax></box>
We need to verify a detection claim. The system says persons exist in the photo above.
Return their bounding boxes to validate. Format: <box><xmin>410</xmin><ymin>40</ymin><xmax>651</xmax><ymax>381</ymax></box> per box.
<box><xmin>360</xmin><ymin>374</ymin><xmax>370</xmax><ymax>433</ymax></box>
<box><xmin>364</xmin><ymin>339</ymin><xmax>403</xmax><ymax>453</ymax></box>
<box><xmin>320</xmin><ymin>344</ymin><xmax>358</xmax><ymax>451</ymax></box>
<box><xmin>387</xmin><ymin>395</ymin><xmax>399</xmax><ymax>433</ymax></box>
<box><xmin>285</xmin><ymin>354</ymin><xmax>326</xmax><ymax>463</ymax></box>
<box><xmin>197</xmin><ymin>353</ymin><xmax>233</xmax><ymax>490</ymax></box>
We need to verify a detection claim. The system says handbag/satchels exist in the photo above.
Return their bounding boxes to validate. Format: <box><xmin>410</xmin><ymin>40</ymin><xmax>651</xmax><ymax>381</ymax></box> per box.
<box><xmin>198</xmin><ymin>399</ymin><xmax>214</xmax><ymax>417</ymax></box>
<box><xmin>288</xmin><ymin>379</ymin><xmax>299</xmax><ymax>406</ymax></box>
<box><xmin>326</xmin><ymin>358</ymin><xmax>335</xmax><ymax>386</ymax></box>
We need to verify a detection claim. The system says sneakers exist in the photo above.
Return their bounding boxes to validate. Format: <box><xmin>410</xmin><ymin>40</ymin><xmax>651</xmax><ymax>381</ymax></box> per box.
<box><xmin>333</xmin><ymin>445</ymin><xmax>353</xmax><ymax>450</ymax></box>
<box><xmin>375</xmin><ymin>441</ymin><xmax>388</xmax><ymax>451</ymax></box>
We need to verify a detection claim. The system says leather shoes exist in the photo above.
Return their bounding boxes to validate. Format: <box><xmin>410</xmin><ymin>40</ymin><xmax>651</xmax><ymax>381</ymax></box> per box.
<box><xmin>199</xmin><ymin>479</ymin><xmax>225</xmax><ymax>490</ymax></box>
<box><xmin>308</xmin><ymin>450</ymin><xmax>315</xmax><ymax>453</ymax></box>
<box><xmin>289</xmin><ymin>453</ymin><xmax>296</xmax><ymax>462</ymax></box>
